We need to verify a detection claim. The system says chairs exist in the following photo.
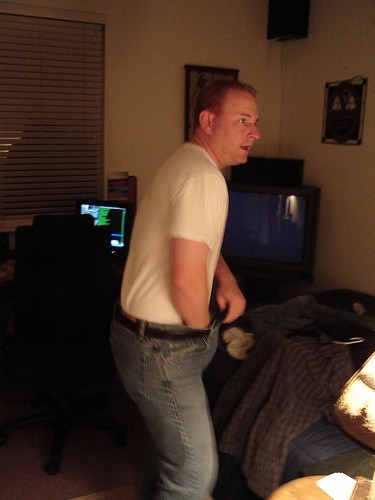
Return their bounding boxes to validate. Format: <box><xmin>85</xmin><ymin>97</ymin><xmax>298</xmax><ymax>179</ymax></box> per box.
<box><xmin>0</xmin><ymin>213</ymin><xmax>130</xmax><ymax>477</ymax></box>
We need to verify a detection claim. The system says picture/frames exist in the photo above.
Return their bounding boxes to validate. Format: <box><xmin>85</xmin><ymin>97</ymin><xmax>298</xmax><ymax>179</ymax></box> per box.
<box><xmin>183</xmin><ymin>64</ymin><xmax>241</xmax><ymax>143</ymax></box>
<box><xmin>320</xmin><ymin>78</ymin><xmax>368</xmax><ymax>146</ymax></box>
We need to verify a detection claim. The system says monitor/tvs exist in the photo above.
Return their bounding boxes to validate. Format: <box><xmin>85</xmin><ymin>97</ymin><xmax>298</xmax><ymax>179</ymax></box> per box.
<box><xmin>75</xmin><ymin>198</ymin><xmax>133</xmax><ymax>253</ymax></box>
<box><xmin>222</xmin><ymin>184</ymin><xmax>321</xmax><ymax>274</ymax></box>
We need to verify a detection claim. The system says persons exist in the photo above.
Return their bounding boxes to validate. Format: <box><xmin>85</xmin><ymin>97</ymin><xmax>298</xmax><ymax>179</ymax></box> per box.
<box><xmin>109</xmin><ymin>79</ymin><xmax>261</xmax><ymax>500</ymax></box>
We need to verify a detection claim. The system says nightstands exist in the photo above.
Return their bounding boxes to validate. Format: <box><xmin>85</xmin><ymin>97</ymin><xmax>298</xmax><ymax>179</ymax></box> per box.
<box><xmin>266</xmin><ymin>474</ymin><xmax>334</xmax><ymax>500</ymax></box>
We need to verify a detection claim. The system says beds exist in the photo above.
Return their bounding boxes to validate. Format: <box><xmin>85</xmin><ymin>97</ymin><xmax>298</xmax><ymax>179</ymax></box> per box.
<box><xmin>201</xmin><ymin>287</ymin><xmax>375</xmax><ymax>500</ymax></box>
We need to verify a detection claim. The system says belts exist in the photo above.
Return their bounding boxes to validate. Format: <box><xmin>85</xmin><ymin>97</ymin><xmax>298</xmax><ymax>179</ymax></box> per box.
<box><xmin>114</xmin><ymin>305</ymin><xmax>210</xmax><ymax>340</ymax></box>
